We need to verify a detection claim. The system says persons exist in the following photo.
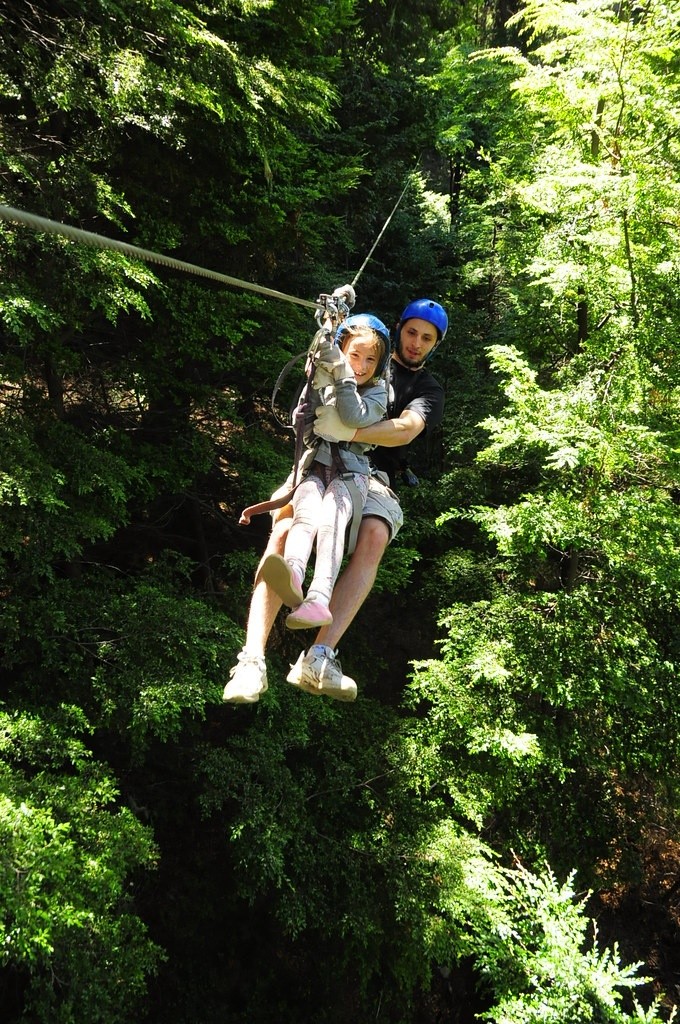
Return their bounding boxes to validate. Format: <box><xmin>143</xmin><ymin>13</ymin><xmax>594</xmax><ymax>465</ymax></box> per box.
<box><xmin>262</xmin><ymin>314</ymin><xmax>395</xmax><ymax>630</ymax></box>
<box><xmin>222</xmin><ymin>299</ymin><xmax>448</xmax><ymax>703</ymax></box>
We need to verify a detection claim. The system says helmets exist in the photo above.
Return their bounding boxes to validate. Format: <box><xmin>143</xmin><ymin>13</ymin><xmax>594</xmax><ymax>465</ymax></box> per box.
<box><xmin>396</xmin><ymin>298</ymin><xmax>449</xmax><ymax>341</ymax></box>
<box><xmin>334</xmin><ymin>313</ymin><xmax>391</xmax><ymax>377</ymax></box>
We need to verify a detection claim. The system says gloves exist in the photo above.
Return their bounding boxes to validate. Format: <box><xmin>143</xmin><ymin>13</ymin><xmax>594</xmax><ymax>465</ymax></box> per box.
<box><xmin>313</xmin><ymin>368</ymin><xmax>334</xmax><ymax>389</ymax></box>
<box><xmin>331</xmin><ymin>284</ymin><xmax>355</xmax><ymax>326</ymax></box>
<box><xmin>312</xmin><ymin>405</ymin><xmax>358</xmax><ymax>443</ymax></box>
<box><xmin>318</xmin><ymin>341</ymin><xmax>355</xmax><ymax>381</ymax></box>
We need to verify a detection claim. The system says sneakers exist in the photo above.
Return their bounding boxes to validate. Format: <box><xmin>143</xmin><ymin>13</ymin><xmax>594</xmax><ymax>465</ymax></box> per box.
<box><xmin>261</xmin><ymin>553</ymin><xmax>303</xmax><ymax>608</ymax></box>
<box><xmin>222</xmin><ymin>645</ymin><xmax>269</xmax><ymax>704</ymax></box>
<box><xmin>285</xmin><ymin>594</ymin><xmax>333</xmax><ymax>629</ymax></box>
<box><xmin>286</xmin><ymin>648</ymin><xmax>357</xmax><ymax>702</ymax></box>
